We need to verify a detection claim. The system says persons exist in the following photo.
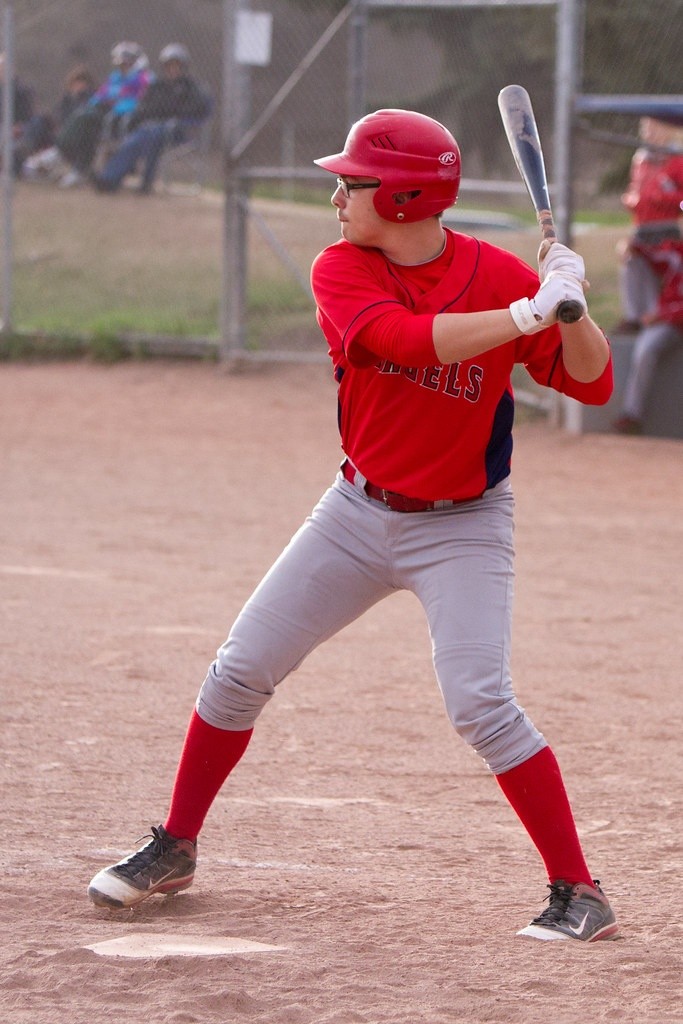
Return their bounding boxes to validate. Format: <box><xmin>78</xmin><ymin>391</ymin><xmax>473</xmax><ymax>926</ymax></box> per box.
<box><xmin>608</xmin><ymin>111</ymin><xmax>683</xmax><ymax>336</ymax></box>
<box><xmin>88</xmin><ymin>107</ymin><xmax>619</xmax><ymax>944</ymax></box>
<box><xmin>94</xmin><ymin>44</ymin><xmax>208</xmax><ymax>193</ymax></box>
<box><xmin>0</xmin><ymin>53</ymin><xmax>32</xmax><ymax>134</ymax></box>
<box><xmin>10</xmin><ymin>41</ymin><xmax>101</xmax><ymax>176</ymax></box>
<box><xmin>612</xmin><ymin>233</ymin><xmax>683</xmax><ymax>435</ymax></box>
<box><xmin>23</xmin><ymin>41</ymin><xmax>157</xmax><ymax>188</ymax></box>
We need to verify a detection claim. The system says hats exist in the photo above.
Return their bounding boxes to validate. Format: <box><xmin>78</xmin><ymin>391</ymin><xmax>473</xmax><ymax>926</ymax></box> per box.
<box><xmin>112</xmin><ymin>42</ymin><xmax>143</xmax><ymax>65</ymax></box>
<box><xmin>159</xmin><ymin>44</ymin><xmax>187</xmax><ymax>65</ymax></box>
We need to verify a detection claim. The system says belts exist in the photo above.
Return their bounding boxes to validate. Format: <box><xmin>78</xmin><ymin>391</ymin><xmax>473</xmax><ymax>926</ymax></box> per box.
<box><xmin>341</xmin><ymin>461</ymin><xmax>484</xmax><ymax>514</ymax></box>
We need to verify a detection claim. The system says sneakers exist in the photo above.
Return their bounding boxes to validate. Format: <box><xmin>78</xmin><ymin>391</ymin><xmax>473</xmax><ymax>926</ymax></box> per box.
<box><xmin>518</xmin><ymin>877</ymin><xmax>620</xmax><ymax>946</ymax></box>
<box><xmin>87</xmin><ymin>826</ymin><xmax>197</xmax><ymax>909</ymax></box>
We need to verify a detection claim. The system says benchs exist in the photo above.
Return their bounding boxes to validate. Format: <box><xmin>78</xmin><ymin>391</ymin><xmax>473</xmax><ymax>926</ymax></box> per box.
<box><xmin>151</xmin><ymin>133</ymin><xmax>210</xmax><ymax>195</ymax></box>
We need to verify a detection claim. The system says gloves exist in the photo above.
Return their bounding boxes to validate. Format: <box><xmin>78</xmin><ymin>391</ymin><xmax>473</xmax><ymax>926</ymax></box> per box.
<box><xmin>510</xmin><ymin>241</ymin><xmax>588</xmax><ymax>336</ymax></box>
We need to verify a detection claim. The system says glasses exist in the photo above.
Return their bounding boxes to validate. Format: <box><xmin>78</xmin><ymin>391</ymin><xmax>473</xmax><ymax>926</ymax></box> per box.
<box><xmin>333</xmin><ymin>175</ymin><xmax>378</xmax><ymax>196</ymax></box>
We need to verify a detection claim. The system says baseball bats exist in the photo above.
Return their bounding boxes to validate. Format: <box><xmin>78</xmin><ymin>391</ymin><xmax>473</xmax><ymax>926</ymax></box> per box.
<box><xmin>497</xmin><ymin>82</ymin><xmax>585</xmax><ymax>325</ymax></box>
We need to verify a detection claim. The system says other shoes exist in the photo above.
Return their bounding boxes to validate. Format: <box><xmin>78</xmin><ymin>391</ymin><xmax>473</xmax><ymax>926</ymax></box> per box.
<box><xmin>607</xmin><ymin>415</ymin><xmax>641</xmax><ymax>434</ymax></box>
<box><xmin>613</xmin><ymin>321</ymin><xmax>640</xmax><ymax>333</ymax></box>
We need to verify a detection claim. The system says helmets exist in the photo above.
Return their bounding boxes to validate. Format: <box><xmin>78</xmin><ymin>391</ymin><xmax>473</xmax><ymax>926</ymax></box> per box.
<box><xmin>313</xmin><ymin>106</ymin><xmax>462</xmax><ymax>224</ymax></box>
